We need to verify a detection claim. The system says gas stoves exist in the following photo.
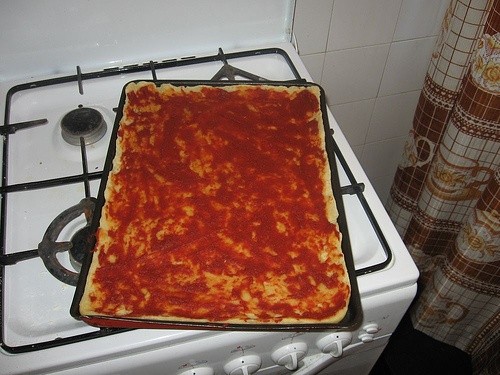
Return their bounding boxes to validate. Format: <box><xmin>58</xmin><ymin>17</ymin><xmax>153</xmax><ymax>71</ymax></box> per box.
<box><xmin>1</xmin><ymin>1</ymin><xmax>421</xmax><ymax>375</ymax></box>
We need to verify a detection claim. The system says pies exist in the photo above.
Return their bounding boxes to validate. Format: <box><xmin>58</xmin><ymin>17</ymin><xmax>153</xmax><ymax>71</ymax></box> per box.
<box><xmin>79</xmin><ymin>80</ymin><xmax>352</xmax><ymax>325</ymax></box>
